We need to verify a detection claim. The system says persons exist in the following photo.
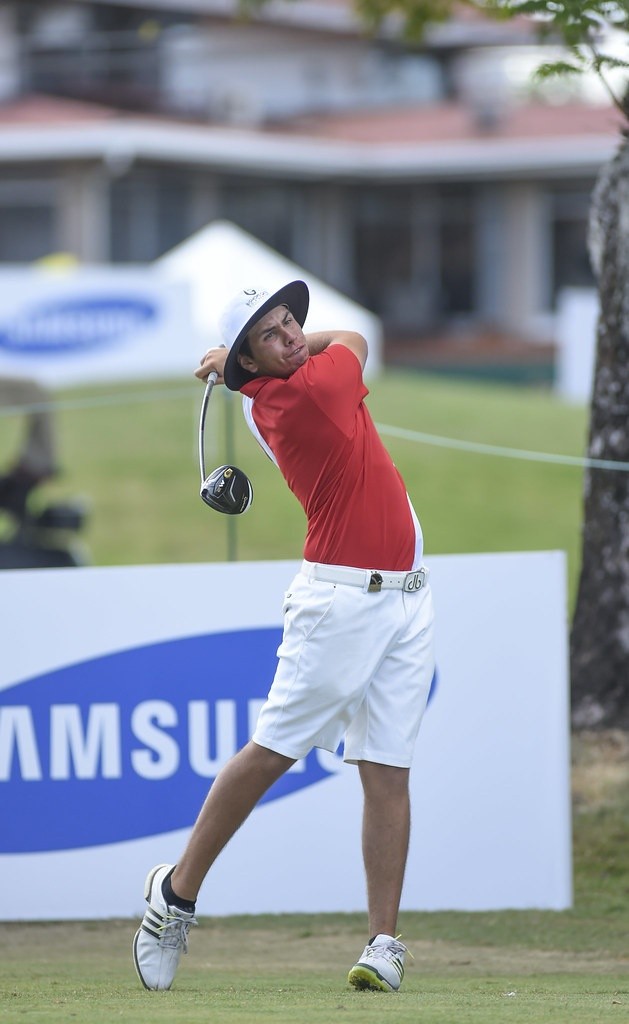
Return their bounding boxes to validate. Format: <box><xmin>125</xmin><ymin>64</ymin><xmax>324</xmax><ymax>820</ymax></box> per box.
<box><xmin>135</xmin><ymin>277</ymin><xmax>440</xmax><ymax>999</ymax></box>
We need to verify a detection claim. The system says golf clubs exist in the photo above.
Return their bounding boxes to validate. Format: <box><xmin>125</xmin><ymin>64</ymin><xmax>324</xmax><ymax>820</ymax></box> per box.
<box><xmin>197</xmin><ymin>344</ymin><xmax>254</xmax><ymax>516</ymax></box>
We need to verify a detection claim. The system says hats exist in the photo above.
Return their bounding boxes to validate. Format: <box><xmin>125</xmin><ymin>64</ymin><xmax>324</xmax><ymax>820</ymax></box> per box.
<box><xmin>220</xmin><ymin>278</ymin><xmax>310</xmax><ymax>392</ymax></box>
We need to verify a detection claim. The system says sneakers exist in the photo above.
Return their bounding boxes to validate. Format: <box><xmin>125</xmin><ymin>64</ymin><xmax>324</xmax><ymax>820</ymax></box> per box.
<box><xmin>131</xmin><ymin>864</ymin><xmax>199</xmax><ymax>991</ymax></box>
<box><xmin>346</xmin><ymin>933</ymin><xmax>415</xmax><ymax>991</ymax></box>
